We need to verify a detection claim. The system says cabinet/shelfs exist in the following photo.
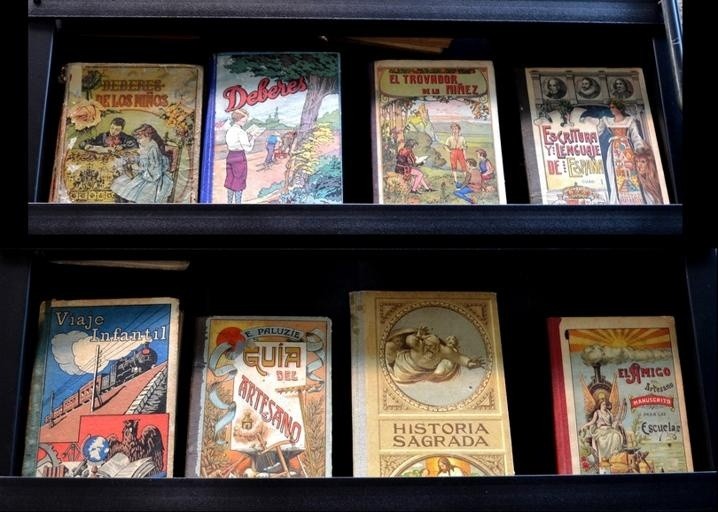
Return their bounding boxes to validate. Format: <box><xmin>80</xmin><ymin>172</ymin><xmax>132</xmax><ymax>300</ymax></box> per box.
<box><xmin>0</xmin><ymin>0</ymin><xmax>718</xmax><ymax>509</ymax></box>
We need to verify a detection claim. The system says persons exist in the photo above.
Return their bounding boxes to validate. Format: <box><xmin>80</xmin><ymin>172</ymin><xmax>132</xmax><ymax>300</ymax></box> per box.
<box><xmin>437</xmin><ymin>457</ymin><xmax>464</xmax><ymax>477</ymax></box>
<box><xmin>223</xmin><ymin>110</ymin><xmax>256</xmax><ymax>204</ymax></box>
<box><xmin>610</xmin><ymin>79</ymin><xmax>633</xmax><ymax>99</ymax></box>
<box><xmin>110</xmin><ymin>123</ymin><xmax>174</xmax><ymax>204</ymax></box>
<box><xmin>545</xmin><ymin>78</ymin><xmax>565</xmax><ymax>99</ymax></box>
<box><xmin>446</xmin><ymin>123</ymin><xmax>495</xmax><ymax>205</ymax></box>
<box><xmin>395</xmin><ymin>138</ymin><xmax>433</xmax><ymax>194</ymax></box>
<box><xmin>386</xmin><ymin>326</ymin><xmax>486</xmax><ymax>384</ymax></box>
<box><xmin>596</xmin><ymin>99</ymin><xmax>650</xmax><ymax>206</ymax></box>
<box><xmin>579</xmin><ymin>399</ymin><xmax>625</xmax><ymax>472</ymax></box>
<box><xmin>578</xmin><ymin>78</ymin><xmax>599</xmax><ymax>99</ymax></box>
<box><xmin>79</xmin><ymin>116</ymin><xmax>137</xmax><ymax>154</ymax></box>
<box><xmin>262</xmin><ymin>131</ymin><xmax>297</xmax><ymax>168</ymax></box>
